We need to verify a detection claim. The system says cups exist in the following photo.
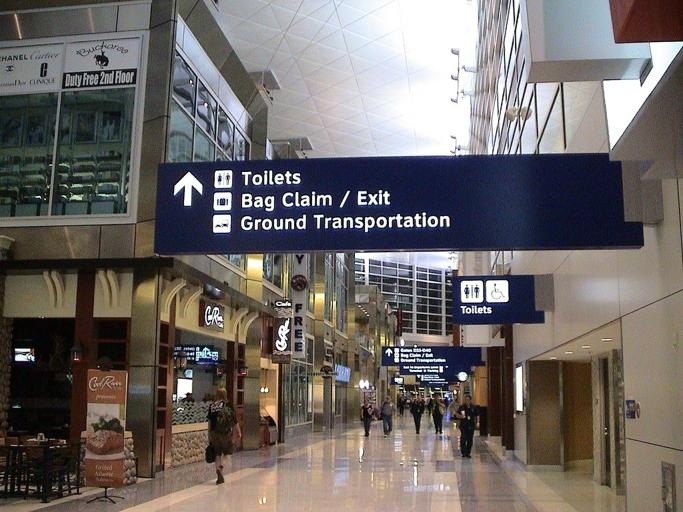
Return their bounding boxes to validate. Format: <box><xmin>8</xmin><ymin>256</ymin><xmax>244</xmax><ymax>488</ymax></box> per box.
<box><xmin>460</xmin><ymin>410</ymin><xmax>466</xmax><ymax>420</ymax></box>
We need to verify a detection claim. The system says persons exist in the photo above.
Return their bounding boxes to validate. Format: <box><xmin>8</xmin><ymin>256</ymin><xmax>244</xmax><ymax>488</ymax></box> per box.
<box><xmin>398</xmin><ymin>394</ymin><xmax>407</xmax><ymax>415</ymax></box>
<box><xmin>453</xmin><ymin>394</ymin><xmax>479</xmax><ymax>458</ymax></box>
<box><xmin>360</xmin><ymin>399</ymin><xmax>372</xmax><ymax>436</ymax></box>
<box><xmin>430</xmin><ymin>392</ymin><xmax>445</xmax><ymax>434</ymax></box>
<box><xmin>410</xmin><ymin>394</ymin><xmax>424</xmax><ymax>434</ymax></box>
<box><xmin>181</xmin><ymin>392</ymin><xmax>194</xmax><ymax>418</ymax></box>
<box><xmin>379</xmin><ymin>395</ymin><xmax>396</xmax><ymax>437</ymax></box>
<box><xmin>205</xmin><ymin>387</ymin><xmax>241</xmax><ymax>484</ymax></box>
<box><xmin>407</xmin><ymin>392</ymin><xmax>459</xmax><ymax>418</ymax></box>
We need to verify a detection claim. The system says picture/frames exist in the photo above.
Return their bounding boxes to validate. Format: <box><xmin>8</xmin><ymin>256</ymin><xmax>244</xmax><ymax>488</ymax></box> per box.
<box><xmin>0</xmin><ymin>108</ymin><xmax>124</xmax><ymax>149</ymax></box>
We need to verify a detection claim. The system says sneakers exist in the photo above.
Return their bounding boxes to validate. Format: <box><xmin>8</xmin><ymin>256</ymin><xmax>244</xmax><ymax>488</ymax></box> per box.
<box><xmin>216</xmin><ymin>468</ymin><xmax>224</xmax><ymax>484</ymax></box>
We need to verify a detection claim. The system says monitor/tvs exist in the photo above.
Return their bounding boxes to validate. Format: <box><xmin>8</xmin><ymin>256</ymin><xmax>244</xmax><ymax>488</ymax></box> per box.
<box><xmin>12</xmin><ymin>345</ymin><xmax>36</xmax><ymax>365</ymax></box>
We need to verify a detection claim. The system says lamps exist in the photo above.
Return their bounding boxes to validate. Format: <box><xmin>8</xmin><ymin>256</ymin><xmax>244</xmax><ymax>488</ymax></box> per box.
<box><xmin>169</xmin><ymin>344</ymin><xmax>188</xmax><ymax>372</ymax></box>
<box><xmin>69</xmin><ymin>338</ymin><xmax>87</xmax><ymax>366</ymax></box>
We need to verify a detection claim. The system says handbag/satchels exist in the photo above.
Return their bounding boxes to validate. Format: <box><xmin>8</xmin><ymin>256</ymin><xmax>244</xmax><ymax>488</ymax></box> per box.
<box><xmin>205</xmin><ymin>446</ymin><xmax>215</xmax><ymax>463</ymax></box>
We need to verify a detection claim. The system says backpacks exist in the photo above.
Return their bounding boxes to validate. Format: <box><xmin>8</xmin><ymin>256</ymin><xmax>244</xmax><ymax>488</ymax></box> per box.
<box><xmin>216</xmin><ymin>407</ymin><xmax>235</xmax><ymax>433</ymax></box>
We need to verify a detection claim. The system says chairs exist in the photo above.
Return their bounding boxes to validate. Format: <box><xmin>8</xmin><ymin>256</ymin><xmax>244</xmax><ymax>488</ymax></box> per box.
<box><xmin>0</xmin><ymin>435</ymin><xmax>39</xmax><ymax>499</ymax></box>
